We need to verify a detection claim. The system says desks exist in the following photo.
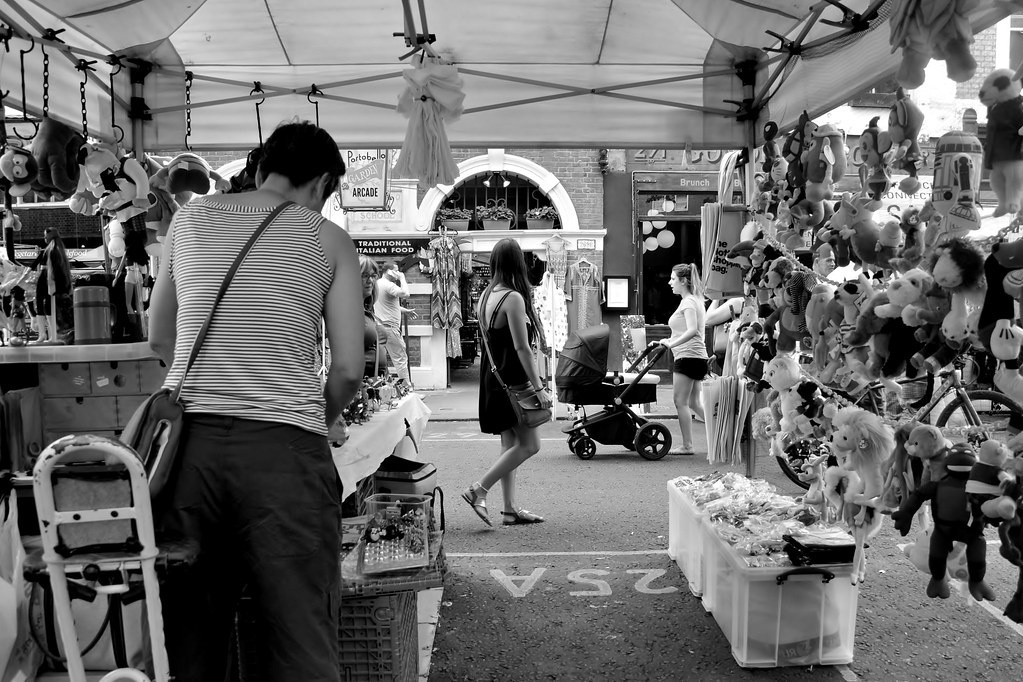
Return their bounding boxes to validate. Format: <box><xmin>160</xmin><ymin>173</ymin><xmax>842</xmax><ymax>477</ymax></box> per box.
<box><xmin>329</xmin><ymin>391</ymin><xmax>433</xmax><ymax>506</ymax></box>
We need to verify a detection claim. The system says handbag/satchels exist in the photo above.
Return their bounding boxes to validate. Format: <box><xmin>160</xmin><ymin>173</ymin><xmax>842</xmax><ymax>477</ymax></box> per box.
<box><xmin>505</xmin><ymin>385</ymin><xmax>552</xmax><ymax>428</ymax></box>
<box><xmin>106</xmin><ymin>388</ymin><xmax>185</xmax><ymax>500</ymax></box>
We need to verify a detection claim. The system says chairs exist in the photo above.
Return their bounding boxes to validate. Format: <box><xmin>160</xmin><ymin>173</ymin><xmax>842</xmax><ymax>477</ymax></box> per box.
<box><xmin>19</xmin><ymin>430</ymin><xmax>173</xmax><ymax>682</ymax></box>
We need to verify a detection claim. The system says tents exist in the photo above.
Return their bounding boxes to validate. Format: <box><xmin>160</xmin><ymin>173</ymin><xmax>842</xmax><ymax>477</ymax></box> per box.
<box><xmin>0</xmin><ymin>0</ymin><xmax>1023</xmax><ymax>162</ymax></box>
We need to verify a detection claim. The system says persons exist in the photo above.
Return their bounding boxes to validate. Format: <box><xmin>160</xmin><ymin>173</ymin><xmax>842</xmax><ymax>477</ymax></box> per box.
<box><xmin>707</xmin><ymin>296</ymin><xmax>746</xmax><ymax>376</ymax></box>
<box><xmin>373</xmin><ymin>260</ymin><xmax>426</xmax><ymax>400</ymax></box>
<box><xmin>358</xmin><ymin>254</ymin><xmax>388</xmax><ymax>378</ymax></box>
<box><xmin>461</xmin><ymin>238</ymin><xmax>553</xmax><ymax>528</ymax></box>
<box><xmin>648</xmin><ymin>263</ymin><xmax>710</xmax><ymax>454</ymax></box>
<box><xmin>147</xmin><ymin>123</ymin><xmax>365</xmax><ymax>682</ymax></box>
<box><xmin>35</xmin><ymin>227</ymin><xmax>73</xmax><ymax>344</ymax></box>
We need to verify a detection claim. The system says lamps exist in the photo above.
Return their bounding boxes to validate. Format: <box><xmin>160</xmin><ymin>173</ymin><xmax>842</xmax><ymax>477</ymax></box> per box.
<box><xmin>482</xmin><ymin>172</ymin><xmax>510</xmax><ymax>188</ymax></box>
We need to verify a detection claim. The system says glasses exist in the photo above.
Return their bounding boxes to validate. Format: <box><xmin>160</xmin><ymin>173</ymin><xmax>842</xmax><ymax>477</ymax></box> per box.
<box><xmin>362</xmin><ymin>273</ymin><xmax>377</xmax><ymax>281</ymax></box>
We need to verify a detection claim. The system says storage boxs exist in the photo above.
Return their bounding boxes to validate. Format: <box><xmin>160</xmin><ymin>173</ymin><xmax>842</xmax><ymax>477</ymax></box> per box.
<box><xmin>334</xmin><ymin>588</ymin><xmax>424</xmax><ymax>681</ymax></box>
<box><xmin>666</xmin><ymin>474</ymin><xmax>856</xmax><ymax>669</ymax></box>
<box><xmin>364</xmin><ymin>492</ymin><xmax>432</xmax><ymax>526</ymax></box>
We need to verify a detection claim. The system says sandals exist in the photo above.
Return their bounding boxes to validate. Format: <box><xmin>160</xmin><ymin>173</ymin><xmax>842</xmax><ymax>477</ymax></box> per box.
<box><xmin>501</xmin><ymin>509</ymin><xmax>544</xmax><ymax>525</ymax></box>
<box><xmin>460</xmin><ymin>485</ymin><xmax>492</xmax><ymax>528</ymax></box>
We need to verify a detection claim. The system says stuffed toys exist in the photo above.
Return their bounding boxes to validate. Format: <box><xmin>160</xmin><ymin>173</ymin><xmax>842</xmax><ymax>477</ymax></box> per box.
<box><xmin>0</xmin><ymin>117</ymin><xmax>232</xmax><ymax>271</ymax></box>
<box><xmin>731</xmin><ymin>70</ymin><xmax>1023</xmax><ymax>625</ymax></box>
<box><xmin>889</xmin><ymin>0</ymin><xmax>978</xmax><ymax>90</ymax></box>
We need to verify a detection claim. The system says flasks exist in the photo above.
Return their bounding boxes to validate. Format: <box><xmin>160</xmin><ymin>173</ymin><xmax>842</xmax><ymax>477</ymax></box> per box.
<box><xmin>72</xmin><ymin>286</ymin><xmax>117</xmax><ymax>345</ymax></box>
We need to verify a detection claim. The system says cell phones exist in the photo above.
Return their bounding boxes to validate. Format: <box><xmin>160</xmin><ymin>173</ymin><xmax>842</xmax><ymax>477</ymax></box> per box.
<box><xmin>388</xmin><ymin>270</ymin><xmax>397</xmax><ymax>279</ymax></box>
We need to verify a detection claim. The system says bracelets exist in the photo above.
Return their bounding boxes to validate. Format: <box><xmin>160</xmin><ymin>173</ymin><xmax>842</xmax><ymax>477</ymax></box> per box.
<box><xmin>535</xmin><ymin>388</ymin><xmax>543</xmax><ymax>392</ymax></box>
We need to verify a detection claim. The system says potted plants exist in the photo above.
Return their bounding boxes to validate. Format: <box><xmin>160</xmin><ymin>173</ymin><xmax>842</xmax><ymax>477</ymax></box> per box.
<box><xmin>476</xmin><ymin>205</ymin><xmax>515</xmax><ymax>230</ymax></box>
<box><xmin>436</xmin><ymin>207</ymin><xmax>474</xmax><ymax>230</ymax></box>
<box><xmin>522</xmin><ymin>205</ymin><xmax>558</xmax><ymax>229</ymax></box>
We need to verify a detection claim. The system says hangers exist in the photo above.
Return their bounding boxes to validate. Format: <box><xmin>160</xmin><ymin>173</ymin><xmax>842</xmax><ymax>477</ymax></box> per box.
<box><xmin>434</xmin><ymin>230</ymin><xmax>449</xmax><ymax>243</ymax></box>
<box><xmin>541</xmin><ymin>232</ymin><xmax>573</xmax><ymax>245</ymax></box>
<box><xmin>577</xmin><ymin>253</ymin><xmax>591</xmax><ymax>270</ymax></box>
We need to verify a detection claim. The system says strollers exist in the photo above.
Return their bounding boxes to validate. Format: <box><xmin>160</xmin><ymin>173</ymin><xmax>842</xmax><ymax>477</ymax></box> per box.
<box><xmin>555</xmin><ymin>323</ymin><xmax>672</xmax><ymax>460</ymax></box>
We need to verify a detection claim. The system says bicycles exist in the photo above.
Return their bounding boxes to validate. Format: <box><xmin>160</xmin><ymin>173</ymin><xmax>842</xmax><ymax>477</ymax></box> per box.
<box><xmin>775</xmin><ymin>343</ymin><xmax>1023</xmax><ymax>492</ymax></box>
